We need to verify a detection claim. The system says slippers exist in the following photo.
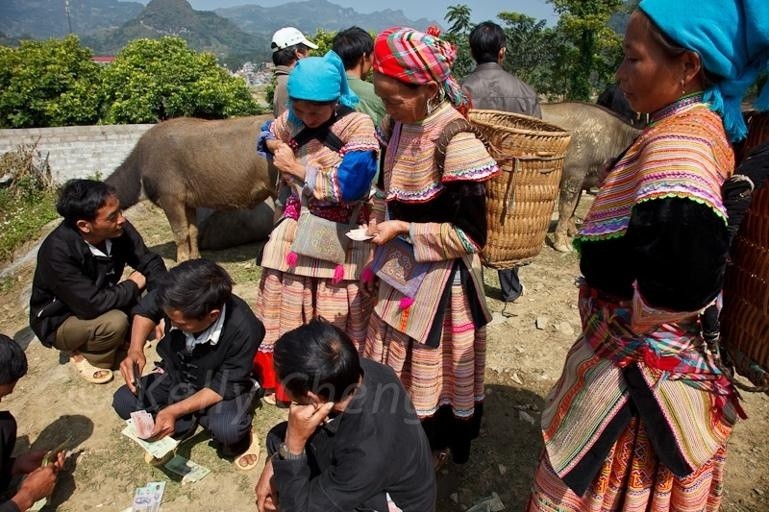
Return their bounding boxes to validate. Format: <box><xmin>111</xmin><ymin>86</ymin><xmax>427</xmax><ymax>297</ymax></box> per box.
<box><xmin>144</xmin><ymin>445</ymin><xmax>179</xmax><ymax>466</ymax></box>
<box><xmin>68</xmin><ymin>354</ymin><xmax>114</xmax><ymax>383</ymax></box>
<box><xmin>233</xmin><ymin>426</ymin><xmax>260</xmax><ymax>472</ymax></box>
<box><xmin>117</xmin><ymin>339</ymin><xmax>151</xmax><ymax>351</ymax></box>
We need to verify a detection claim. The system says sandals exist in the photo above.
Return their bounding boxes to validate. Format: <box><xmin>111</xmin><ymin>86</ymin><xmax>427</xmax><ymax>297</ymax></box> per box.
<box><xmin>430</xmin><ymin>445</ymin><xmax>456</xmax><ymax>479</ymax></box>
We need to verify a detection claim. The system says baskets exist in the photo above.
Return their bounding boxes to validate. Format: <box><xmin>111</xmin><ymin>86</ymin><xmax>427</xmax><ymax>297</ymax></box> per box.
<box><xmin>465</xmin><ymin>108</ymin><xmax>572</xmax><ymax>271</ymax></box>
<box><xmin>720</xmin><ymin>152</ymin><xmax>769</xmax><ymax>400</ymax></box>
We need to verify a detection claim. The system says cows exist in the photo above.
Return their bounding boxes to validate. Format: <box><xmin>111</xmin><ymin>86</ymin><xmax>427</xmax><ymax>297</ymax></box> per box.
<box><xmin>543</xmin><ymin>101</ymin><xmax>648</xmax><ymax>254</ymax></box>
<box><xmin>102</xmin><ymin>114</ymin><xmax>285</xmax><ymax>265</ymax></box>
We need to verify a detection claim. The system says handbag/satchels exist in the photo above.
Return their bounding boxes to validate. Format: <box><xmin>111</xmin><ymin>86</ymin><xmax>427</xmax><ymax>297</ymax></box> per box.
<box><xmin>290</xmin><ymin>212</ymin><xmax>358</xmax><ymax>266</ymax></box>
<box><xmin>369</xmin><ymin>236</ymin><xmax>432</xmax><ymax>298</ymax></box>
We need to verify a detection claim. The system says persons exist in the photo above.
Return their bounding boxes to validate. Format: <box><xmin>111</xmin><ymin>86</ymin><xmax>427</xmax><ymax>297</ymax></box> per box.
<box><xmin>524</xmin><ymin>0</ymin><xmax>769</xmax><ymax>512</ymax></box>
<box><xmin>330</xmin><ymin>23</ymin><xmax>389</xmax><ymax>135</ymax></box>
<box><xmin>355</xmin><ymin>26</ymin><xmax>501</xmax><ymax>479</ymax></box>
<box><xmin>111</xmin><ymin>255</ymin><xmax>266</xmax><ymax>471</ymax></box>
<box><xmin>251</xmin><ymin>58</ymin><xmax>381</xmax><ymax>412</ymax></box>
<box><xmin>271</xmin><ymin>25</ymin><xmax>320</xmax><ymax>125</ymax></box>
<box><xmin>253</xmin><ymin>314</ymin><xmax>439</xmax><ymax>511</ymax></box>
<box><xmin>30</xmin><ymin>177</ymin><xmax>169</xmax><ymax>384</ymax></box>
<box><xmin>1</xmin><ymin>332</ymin><xmax>66</xmax><ymax>512</ymax></box>
<box><xmin>458</xmin><ymin>20</ymin><xmax>542</xmax><ymax>304</ymax></box>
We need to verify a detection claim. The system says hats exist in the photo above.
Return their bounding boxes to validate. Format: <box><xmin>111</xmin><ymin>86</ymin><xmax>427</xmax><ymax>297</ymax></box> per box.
<box><xmin>270</xmin><ymin>27</ymin><xmax>319</xmax><ymax>53</ymax></box>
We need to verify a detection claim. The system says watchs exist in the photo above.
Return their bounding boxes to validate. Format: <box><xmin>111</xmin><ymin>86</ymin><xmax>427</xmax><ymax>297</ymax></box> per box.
<box><xmin>278</xmin><ymin>443</ymin><xmax>306</xmax><ymax>462</ymax></box>
